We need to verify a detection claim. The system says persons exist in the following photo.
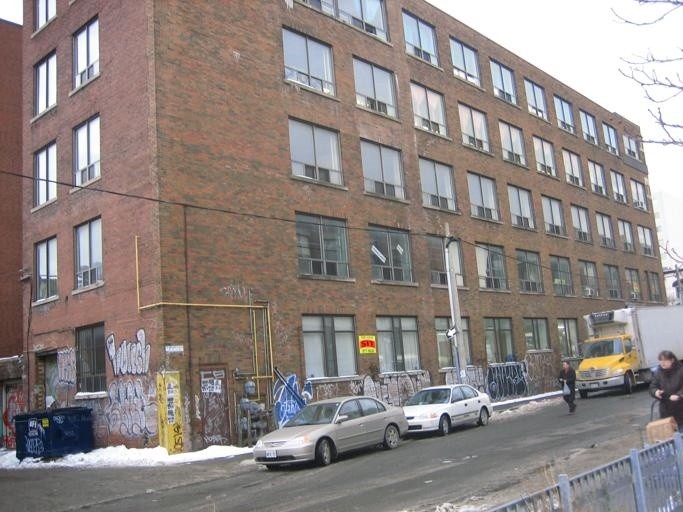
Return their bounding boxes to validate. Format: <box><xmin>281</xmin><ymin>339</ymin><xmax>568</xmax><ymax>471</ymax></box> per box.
<box><xmin>649</xmin><ymin>350</ymin><xmax>683</xmax><ymax>439</ymax></box>
<box><xmin>557</xmin><ymin>360</ymin><xmax>577</xmax><ymax>414</ymax></box>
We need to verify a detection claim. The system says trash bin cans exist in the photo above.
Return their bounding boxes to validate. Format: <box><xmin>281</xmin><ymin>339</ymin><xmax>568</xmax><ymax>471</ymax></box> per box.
<box><xmin>12</xmin><ymin>406</ymin><xmax>93</xmax><ymax>463</ymax></box>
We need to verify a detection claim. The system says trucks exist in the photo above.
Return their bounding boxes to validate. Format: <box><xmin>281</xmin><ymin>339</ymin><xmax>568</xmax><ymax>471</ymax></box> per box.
<box><xmin>570</xmin><ymin>302</ymin><xmax>683</xmax><ymax>400</ymax></box>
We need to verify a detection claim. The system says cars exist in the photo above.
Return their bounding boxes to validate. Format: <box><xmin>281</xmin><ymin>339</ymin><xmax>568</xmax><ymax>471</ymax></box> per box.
<box><xmin>252</xmin><ymin>392</ymin><xmax>410</xmax><ymax>469</ymax></box>
<box><xmin>401</xmin><ymin>383</ymin><xmax>494</xmax><ymax>435</ymax></box>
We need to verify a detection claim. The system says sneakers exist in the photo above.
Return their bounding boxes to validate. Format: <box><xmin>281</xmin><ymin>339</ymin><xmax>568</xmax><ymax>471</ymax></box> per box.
<box><xmin>567</xmin><ymin>404</ymin><xmax>577</xmax><ymax>415</ymax></box>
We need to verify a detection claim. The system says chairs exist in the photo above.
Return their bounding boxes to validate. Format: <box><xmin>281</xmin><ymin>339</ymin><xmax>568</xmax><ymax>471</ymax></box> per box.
<box><xmin>323</xmin><ymin>408</ymin><xmax>332</xmax><ymax>417</ymax></box>
<box><xmin>430</xmin><ymin>392</ymin><xmax>438</xmax><ymax>402</ymax></box>
